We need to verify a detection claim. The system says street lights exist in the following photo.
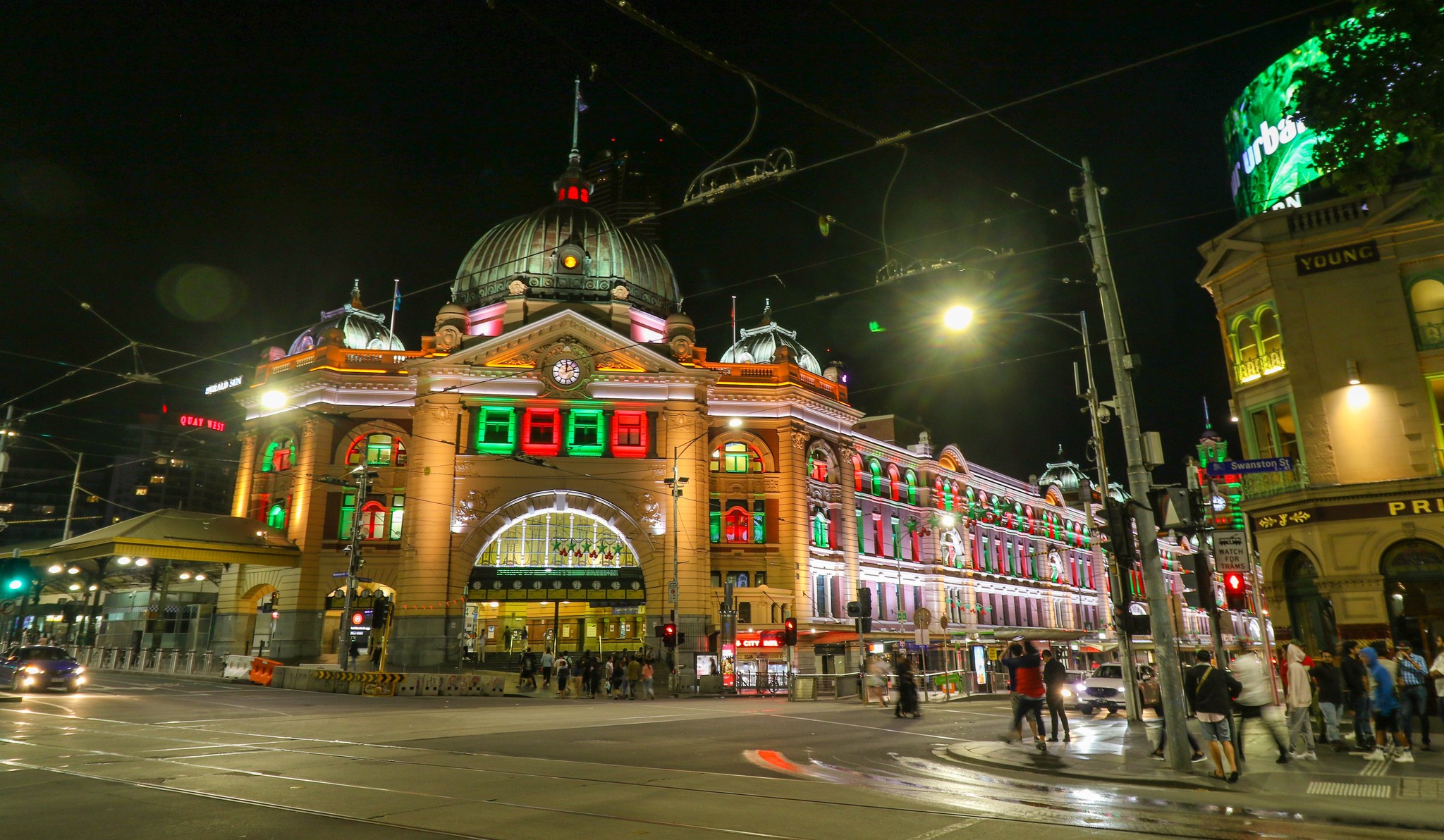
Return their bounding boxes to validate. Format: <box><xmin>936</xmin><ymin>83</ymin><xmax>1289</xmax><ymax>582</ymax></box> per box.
<box><xmin>940</xmin><ymin>306</ymin><xmax>1138</xmax><ymax>702</ymax></box>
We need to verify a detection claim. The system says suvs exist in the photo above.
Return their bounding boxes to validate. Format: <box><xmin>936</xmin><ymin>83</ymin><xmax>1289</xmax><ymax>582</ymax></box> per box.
<box><xmin>1079</xmin><ymin>661</ymin><xmax>1163</xmax><ymax>717</ymax></box>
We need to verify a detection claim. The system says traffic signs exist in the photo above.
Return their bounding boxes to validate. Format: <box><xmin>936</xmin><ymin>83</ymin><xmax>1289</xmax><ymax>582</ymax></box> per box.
<box><xmin>1207</xmin><ymin>458</ymin><xmax>1293</xmax><ymax>475</ymax></box>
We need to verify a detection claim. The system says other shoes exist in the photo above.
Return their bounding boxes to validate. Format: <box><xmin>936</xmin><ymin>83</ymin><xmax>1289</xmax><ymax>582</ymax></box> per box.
<box><xmin>516</xmin><ymin>679</ymin><xmax>655</xmax><ymax>701</ymax></box>
<box><xmin>997</xmin><ymin>735</ymin><xmax>1070</xmax><ymax>751</ymax></box>
<box><xmin>477</xmin><ymin>661</ymin><xmax>485</xmax><ymax>663</ymax></box>
<box><xmin>465</xmin><ymin>657</ymin><xmax>471</xmax><ymax>661</ymax></box>
<box><xmin>1190</xmin><ymin>752</ymin><xmax>1208</xmax><ymax>763</ymax></box>
<box><xmin>882</xmin><ymin>702</ymin><xmax>921</xmax><ymax>719</ymax></box>
<box><xmin>1241</xmin><ymin>733</ymin><xmax>1430</xmax><ymax>763</ymax></box>
<box><xmin>462</xmin><ymin>657</ymin><xmax>464</xmax><ymax>660</ymax></box>
<box><xmin>1146</xmin><ymin>752</ymin><xmax>1165</xmax><ymax>761</ymax></box>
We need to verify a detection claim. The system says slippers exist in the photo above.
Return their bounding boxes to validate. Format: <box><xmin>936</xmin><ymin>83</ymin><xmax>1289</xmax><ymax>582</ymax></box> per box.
<box><xmin>1227</xmin><ymin>771</ymin><xmax>1238</xmax><ymax>783</ymax></box>
<box><xmin>1208</xmin><ymin>769</ymin><xmax>1226</xmax><ymax>781</ymax></box>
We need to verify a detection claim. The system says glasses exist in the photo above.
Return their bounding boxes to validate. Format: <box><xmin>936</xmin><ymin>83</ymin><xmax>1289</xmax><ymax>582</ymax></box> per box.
<box><xmin>1322</xmin><ymin>656</ymin><xmax>1332</xmax><ymax>659</ymax></box>
<box><xmin>1042</xmin><ymin>657</ymin><xmax>1044</xmax><ymax>662</ymax></box>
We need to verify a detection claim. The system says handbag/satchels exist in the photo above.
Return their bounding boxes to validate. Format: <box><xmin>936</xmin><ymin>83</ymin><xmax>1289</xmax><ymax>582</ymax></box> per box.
<box><xmin>640</xmin><ymin>677</ymin><xmax>645</xmax><ymax>683</ymax></box>
<box><xmin>541</xmin><ymin>667</ymin><xmax>543</xmax><ymax>671</ymax></box>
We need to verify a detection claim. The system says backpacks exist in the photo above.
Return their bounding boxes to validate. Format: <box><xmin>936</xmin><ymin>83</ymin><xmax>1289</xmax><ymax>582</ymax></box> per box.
<box><xmin>562</xmin><ymin>656</ymin><xmax>572</xmax><ymax>670</ymax></box>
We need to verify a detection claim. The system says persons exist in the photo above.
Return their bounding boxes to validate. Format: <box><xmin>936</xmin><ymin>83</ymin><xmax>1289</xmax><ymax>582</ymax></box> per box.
<box><xmin>730</xmin><ymin>657</ymin><xmax>735</xmax><ymax>672</ymax></box>
<box><xmin>724</xmin><ymin>659</ymin><xmax>732</xmax><ymax>673</ymax></box>
<box><xmin>349</xmin><ymin>641</ymin><xmax>359</xmax><ymax>671</ymax></box>
<box><xmin>370</xmin><ymin>641</ymin><xmax>382</xmax><ymax>672</ymax></box>
<box><xmin>1086</xmin><ymin>634</ymin><xmax>1444</xmax><ymax>785</ymax></box>
<box><xmin>999</xmin><ymin>641</ymin><xmax>1047</xmax><ymax>751</ymax></box>
<box><xmin>0</xmin><ymin>625</ymin><xmax>66</xmax><ymax>662</ymax></box>
<box><xmin>502</xmin><ymin>625</ymin><xmax>512</xmax><ymax>652</ymax></box>
<box><xmin>520</xmin><ymin>626</ymin><xmax>528</xmax><ymax>652</ymax></box>
<box><xmin>476</xmin><ymin>628</ymin><xmax>487</xmax><ymax>663</ymax></box>
<box><xmin>894</xmin><ymin>657</ymin><xmax>918</xmax><ymax>719</ymax></box>
<box><xmin>710</xmin><ymin>658</ymin><xmax>717</xmax><ymax>675</ymax></box>
<box><xmin>871</xmin><ymin>656</ymin><xmax>888</xmax><ymax>709</ymax></box>
<box><xmin>457</xmin><ymin>628</ymin><xmax>471</xmax><ymax>660</ymax></box>
<box><xmin>519</xmin><ymin>644</ymin><xmax>657</xmax><ymax>702</ymax></box>
<box><xmin>897</xmin><ymin>656</ymin><xmax>922</xmax><ymax>717</ymax></box>
<box><xmin>720</xmin><ymin>659</ymin><xmax>726</xmax><ymax>674</ymax></box>
<box><xmin>1042</xmin><ymin>649</ymin><xmax>1071</xmax><ymax>743</ymax></box>
<box><xmin>998</xmin><ymin>640</ymin><xmax>1040</xmax><ymax>742</ymax></box>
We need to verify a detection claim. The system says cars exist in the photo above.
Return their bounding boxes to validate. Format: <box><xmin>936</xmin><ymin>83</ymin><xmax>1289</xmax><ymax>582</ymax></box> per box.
<box><xmin>0</xmin><ymin>646</ymin><xmax>82</xmax><ymax>694</ymax></box>
<box><xmin>1063</xmin><ymin>669</ymin><xmax>1094</xmax><ymax>709</ymax></box>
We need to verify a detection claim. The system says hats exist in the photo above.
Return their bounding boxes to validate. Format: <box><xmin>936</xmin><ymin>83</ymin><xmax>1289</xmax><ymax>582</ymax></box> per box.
<box><xmin>1395</xmin><ymin>640</ymin><xmax>1411</xmax><ymax>650</ymax></box>
<box><xmin>377</xmin><ymin>642</ymin><xmax>381</xmax><ymax>644</ymax></box>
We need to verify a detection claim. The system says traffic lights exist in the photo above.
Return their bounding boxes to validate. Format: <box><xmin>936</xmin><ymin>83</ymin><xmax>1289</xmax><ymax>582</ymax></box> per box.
<box><xmin>1095</xmin><ymin>495</ymin><xmax>1141</xmax><ymax>571</ymax></box>
<box><xmin>1177</xmin><ymin>552</ymin><xmax>1210</xmax><ymax>609</ymax></box>
<box><xmin>370</xmin><ymin>599</ymin><xmax>386</xmax><ymax>628</ymax></box>
<box><xmin>1114</xmin><ymin>602</ymin><xmax>1132</xmax><ymax>632</ymax></box>
<box><xmin>785</xmin><ymin>617</ymin><xmax>797</xmax><ymax>645</ymax></box>
<box><xmin>661</xmin><ymin>623</ymin><xmax>677</xmax><ymax>647</ymax></box>
<box><xmin>0</xmin><ymin>557</ymin><xmax>32</xmax><ymax>595</ymax></box>
<box><xmin>858</xmin><ymin>588</ymin><xmax>872</xmax><ymax>617</ymax></box>
